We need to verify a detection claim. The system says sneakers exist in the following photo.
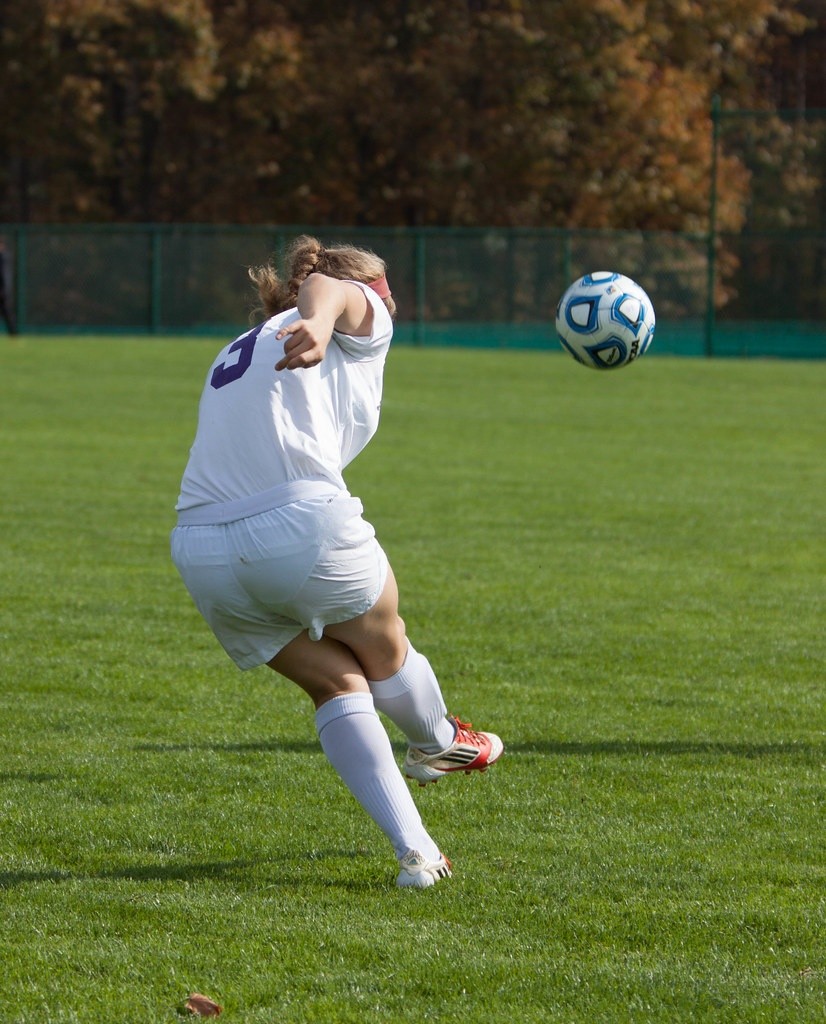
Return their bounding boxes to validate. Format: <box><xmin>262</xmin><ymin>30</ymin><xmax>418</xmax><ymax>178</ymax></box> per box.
<box><xmin>403</xmin><ymin>713</ymin><xmax>504</xmax><ymax>789</ymax></box>
<box><xmin>393</xmin><ymin>852</ymin><xmax>452</xmax><ymax>888</ymax></box>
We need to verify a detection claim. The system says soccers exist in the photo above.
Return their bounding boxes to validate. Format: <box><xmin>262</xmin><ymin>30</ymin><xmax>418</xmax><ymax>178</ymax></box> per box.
<box><xmin>555</xmin><ymin>272</ymin><xmax>655</xmax><ymax>370</ymax></box>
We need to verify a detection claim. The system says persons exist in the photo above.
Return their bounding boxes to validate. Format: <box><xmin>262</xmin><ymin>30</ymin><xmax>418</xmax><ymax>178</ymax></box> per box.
<box><xmin>171</xmin><ymin>235</ymin><xmax>503</xmax><ymax>890</ymax></box>
